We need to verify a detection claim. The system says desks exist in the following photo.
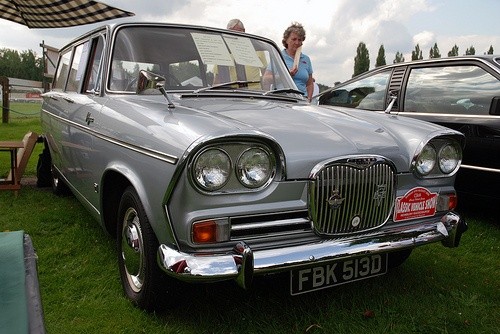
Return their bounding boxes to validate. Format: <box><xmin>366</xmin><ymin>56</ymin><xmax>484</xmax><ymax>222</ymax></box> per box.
<box><xmin>0</xmin><ymin>140</ymin><xmax>25</xmax><ymax>190</ymax></box>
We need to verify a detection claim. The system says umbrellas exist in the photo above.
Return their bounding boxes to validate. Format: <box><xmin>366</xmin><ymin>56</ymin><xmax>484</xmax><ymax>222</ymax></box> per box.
<box><xmin>1</xmin><ymin>1</ymin><xmax>135</xmax><ymax>29</ymax></box>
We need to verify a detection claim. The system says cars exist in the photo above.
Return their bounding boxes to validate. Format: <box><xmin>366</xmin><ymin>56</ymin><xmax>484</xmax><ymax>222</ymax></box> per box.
<box><xmin>39</xmin><ymin>19</ymin><xmax>469</xmax><ymax>315</ymax></box>
<box><xmin>308</xmin><ymin>54</ymin><xmax>500</xmax><ymax>207</ymax></box>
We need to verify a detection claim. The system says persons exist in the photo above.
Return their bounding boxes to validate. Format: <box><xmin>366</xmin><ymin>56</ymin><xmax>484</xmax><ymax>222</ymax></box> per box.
<box><xmin>261</xmin><ymin>21</ymin><xmax>314</xmax><ymax>103</ymax></box>
<box><xmin>334</xmin><ymin>82</ymin><xmax>353</xmax><ymax>105</ymax></box>
<box><xmin>312</xmin><ymin>78</ymin><xmax>320</xmax><ymax>104</ymax></box>
<box><xmin>213</xmin><ymin>18</ymin><xmax>270</xmax><ymax>92</ymax></box>
